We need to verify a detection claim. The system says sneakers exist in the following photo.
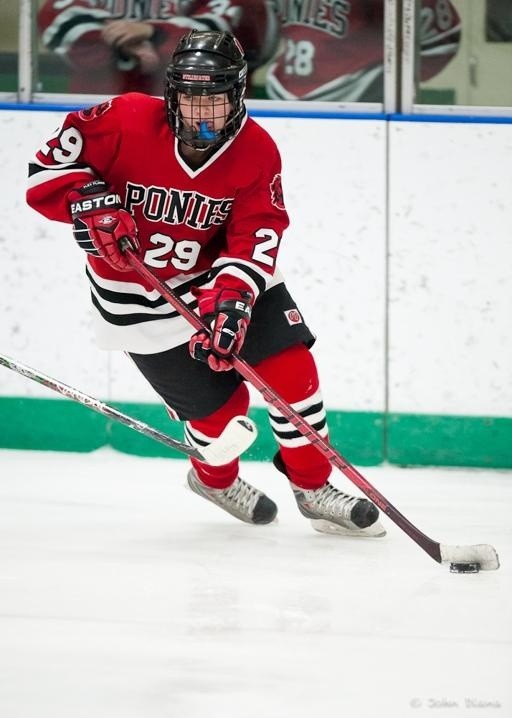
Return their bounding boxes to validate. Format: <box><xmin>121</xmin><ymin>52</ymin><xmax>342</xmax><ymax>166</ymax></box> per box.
<box><xmin>186</xmin><ymin>466</ymin><xmax>278</xmax><ymax>526</ymax></box>
<box><xmin>289</xmin><ymin>480</ymin><xmax>380</xmax><ymax>533</ymax></box>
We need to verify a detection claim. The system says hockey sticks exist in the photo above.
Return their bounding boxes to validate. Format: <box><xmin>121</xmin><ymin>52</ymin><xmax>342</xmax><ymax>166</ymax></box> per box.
<box><xmin>0</xmin><ymin>356</ymin><xmax>258</xmax><ymax>468</ymax></box>
<box><xmin>117</xmin><ymin>246</ymin><xmax>499</xmax><ymax>575</ymax></box>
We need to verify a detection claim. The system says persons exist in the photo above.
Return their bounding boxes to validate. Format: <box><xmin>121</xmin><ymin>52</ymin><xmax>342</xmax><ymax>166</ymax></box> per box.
<box><xmin>21</xmin><ymin>30</ymin><xmax>382</xmax><ymax>530</ymax></box>
<box><xmin>34</xmin><ymin>0</ymin><xmax>270</xmax><ymax>100</ymax></box>
<box><xmin>264</xmin><ymin>0</ymin><xmax>462</xmax><ymax>102</ymax></box>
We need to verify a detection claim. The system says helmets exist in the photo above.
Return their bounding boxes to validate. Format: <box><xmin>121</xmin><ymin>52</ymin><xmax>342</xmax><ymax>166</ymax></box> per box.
<box><xmin>162</xmin><ymin>27</ymin><xmax>248</xmax><ymax>150</ymax></box>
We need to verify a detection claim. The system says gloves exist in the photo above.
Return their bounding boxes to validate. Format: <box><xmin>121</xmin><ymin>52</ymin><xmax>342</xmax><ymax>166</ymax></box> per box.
<box><xmin>186</xmin><ymin>282</ymin><xmax>256</xmax><ymax>374</ymax></box>
<box><xmin>67</xmin><ymin>178</ymin><xmax>143</xmax><ymax>274</ymax></box>
